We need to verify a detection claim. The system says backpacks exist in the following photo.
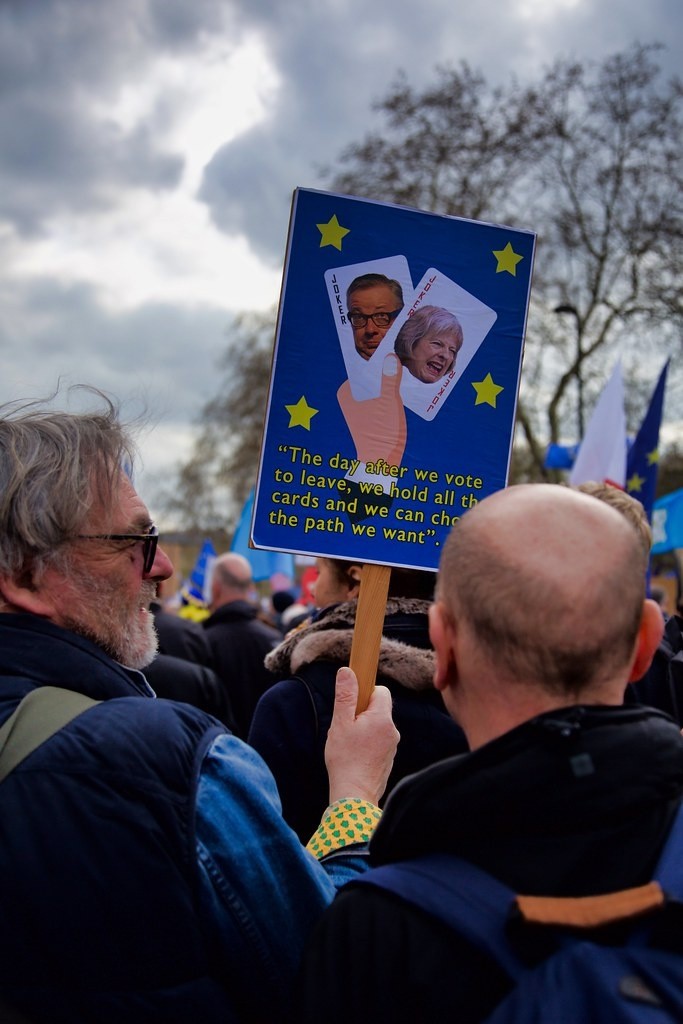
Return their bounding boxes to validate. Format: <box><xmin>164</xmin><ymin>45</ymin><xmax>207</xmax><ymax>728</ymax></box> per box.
<box><xmin>343</xmin><ymin>820</ymin><xmax>683</xmax><ymax>1024</ymax></box>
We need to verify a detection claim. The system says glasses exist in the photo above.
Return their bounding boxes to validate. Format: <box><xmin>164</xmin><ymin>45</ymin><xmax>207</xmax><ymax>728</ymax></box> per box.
<box><xmin>75</xmin><ymin>526</ymin><xmax>159</xmax><ymax>572</ymax></box>
<box><xmin>346</xmin><ymin>307</ymin><xmax>402</xmax><ymax>328</ymax></box>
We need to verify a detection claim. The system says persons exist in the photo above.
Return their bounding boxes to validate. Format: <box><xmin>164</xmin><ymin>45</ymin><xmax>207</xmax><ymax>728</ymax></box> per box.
<box><xmin>394</xmin><ymin>306</ymin><xmax>463</xmax><ymax>384</ymax></box>
<box><xmin>347</xmin><ymin>274</ymin><xmax>404</xmax><ymax>360</ymax></box>
<box><xmin>0</xmin><ymin>415</ymin><xmax>682</xmax><ymax>1024</ymax></box>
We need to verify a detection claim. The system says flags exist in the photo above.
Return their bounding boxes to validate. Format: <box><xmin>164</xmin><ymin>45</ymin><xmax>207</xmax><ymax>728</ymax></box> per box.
<box><xmin>542</xmin><ymin>356</ymin><xmax>683</xmax><ymax>600</ymax></box>
<box><xmin>180</xmin><ymin>539</ymin><xmax>218</xmax><ymax>607</ymax></box>
<box><xmin>231</xmin><ymin>488</ymin><xmax>294</xmax><ymax>584</ymax></box>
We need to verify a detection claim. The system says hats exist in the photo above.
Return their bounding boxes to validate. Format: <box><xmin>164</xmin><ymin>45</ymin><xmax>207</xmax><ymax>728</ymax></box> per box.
<box><xmin>273</xmin><ymin>593</ymin><xmax>295</xmax><ymax>612</ymax></box>
